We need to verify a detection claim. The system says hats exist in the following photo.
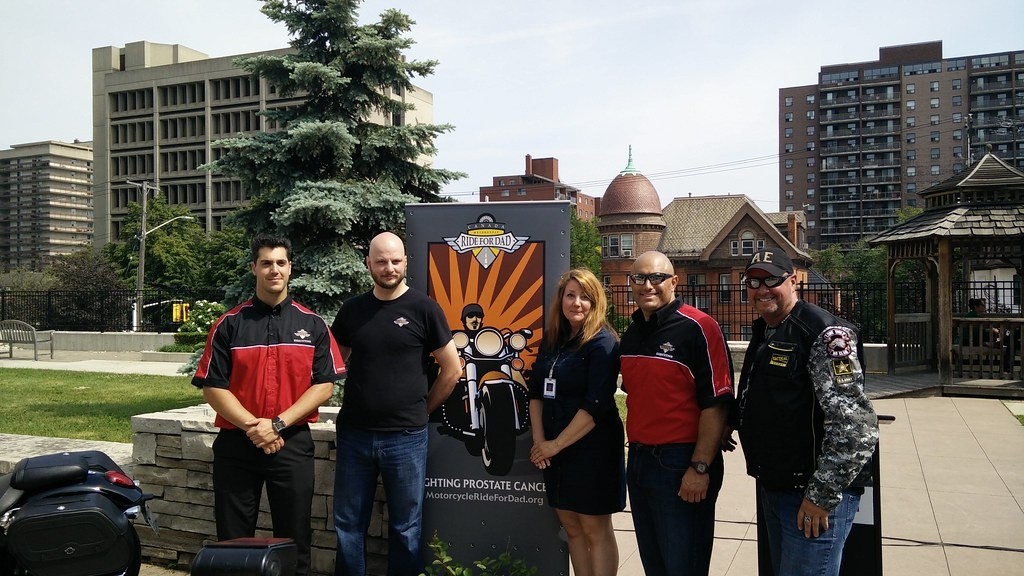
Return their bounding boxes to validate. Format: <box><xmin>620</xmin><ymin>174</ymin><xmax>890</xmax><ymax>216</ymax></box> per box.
<box><xmin>744</xmin><ymin>248</ymin><xmax>795</xmax><ymax>273</ymax></box>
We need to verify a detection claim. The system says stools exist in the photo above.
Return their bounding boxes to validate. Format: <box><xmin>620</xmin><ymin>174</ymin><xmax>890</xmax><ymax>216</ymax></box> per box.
<box><xmin>952</xmin><ymin>345</ymin><xmax>1007</xmax><ymax>379</ymax></box>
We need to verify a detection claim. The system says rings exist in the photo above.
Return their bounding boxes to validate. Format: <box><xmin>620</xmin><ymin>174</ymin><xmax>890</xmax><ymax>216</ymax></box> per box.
<box><xmin>805</xmin><ymin>516</ymin><xmax>811</xmax><ymax>522</ymax></box>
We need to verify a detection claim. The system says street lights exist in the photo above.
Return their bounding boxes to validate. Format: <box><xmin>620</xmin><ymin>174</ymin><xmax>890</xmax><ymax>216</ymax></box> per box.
<box><xmin>137</xmin><ymin>216</ymin><xmax>194</xmax><ymax>331</ymax></box>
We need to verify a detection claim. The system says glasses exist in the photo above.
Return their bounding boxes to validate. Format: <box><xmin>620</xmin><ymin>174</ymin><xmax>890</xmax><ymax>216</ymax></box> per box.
<box><xmin>745</xmin><ymin>274</ymin><xmax>795</xmax><ymax>288</ymax></box>
<box><xmin>626</xmin><ymin>272</ymin><xmax>674</xmax><ymax>286</ymax></box>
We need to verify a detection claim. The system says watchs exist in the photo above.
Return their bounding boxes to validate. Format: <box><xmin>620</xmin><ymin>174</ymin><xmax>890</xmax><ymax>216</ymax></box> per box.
<box><xmin>690</xmin><ymin>461</ymin><xmax>709</xmax><ymax>475</ymax></box>
<box><xmin>272</xmin><ymin>417</ymin><xmax>286</xmax><ymax>432</ymax></box>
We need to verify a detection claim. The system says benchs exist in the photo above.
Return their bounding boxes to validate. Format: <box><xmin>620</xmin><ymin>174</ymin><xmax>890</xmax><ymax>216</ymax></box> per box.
<box><xmin>0</xmin><ymin>320</ymin><xmax>54</xmax><ymax>361</ymax></box>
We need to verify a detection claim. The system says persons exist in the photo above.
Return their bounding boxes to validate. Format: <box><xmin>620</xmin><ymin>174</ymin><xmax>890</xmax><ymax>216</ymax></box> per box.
<box><xmin>328</xmin><ymin>232</ymin><xmax>462</xmax><ymax>576</ymax></box>
<box><xmin>191</xmin><ymin>235</ymin><xmax>347</xmax><ymax>576</ymax></box>
<box><xmin>528</xmin><ymin>268</ymin><xmax>627</xmax><ymax>576</ymax></box>
<box><xmin>722</xmin><ymin>248</ymin><xmax>879</xmax><ymax>576</ymax></box>
<box><xmin>547</xmin><ymin>384</ymin><xmax>553</xmax><ymax>391</ymax></box>
<box><xmin>617</xmin><ymin>250</ymin><xmax>735</xmax><ymax>576</ymax></box>
<box><xmin>961</xmin><ymin>299</ymin><xmax>997</xmax><ymax>346</ymax></box>
